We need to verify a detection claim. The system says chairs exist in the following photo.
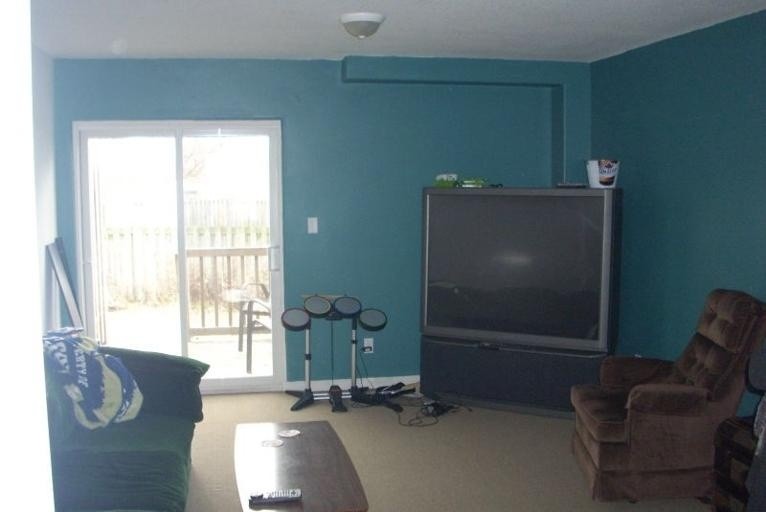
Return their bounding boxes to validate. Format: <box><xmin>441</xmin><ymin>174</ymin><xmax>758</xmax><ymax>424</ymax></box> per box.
<box><xmin>570</xmin><ymin>288</ymin><xmax>766</xmax><ymax>502</ymax></box>
<box><xmin>43</xmin><ymin>326</ymin><xmax>210</xmax><ymax>512</ymax></box>
<box><xmin>223</xmin><ymin>289</ymin><xmax>272</xmax><ymax>373</ymax></box>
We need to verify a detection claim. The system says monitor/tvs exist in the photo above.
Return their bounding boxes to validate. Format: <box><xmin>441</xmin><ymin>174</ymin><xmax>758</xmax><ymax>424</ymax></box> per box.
<box><xmin>421</xmin><ymin>186</ymin><xmax>621</xmax><ymax>354</ymax></box>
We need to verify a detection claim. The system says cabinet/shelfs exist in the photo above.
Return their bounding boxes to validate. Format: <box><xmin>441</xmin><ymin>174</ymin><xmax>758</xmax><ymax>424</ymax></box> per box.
<box><xmin>419</xmin><ymin>334</ymin><xmax>610</xmax><ymax>420</ymax></box>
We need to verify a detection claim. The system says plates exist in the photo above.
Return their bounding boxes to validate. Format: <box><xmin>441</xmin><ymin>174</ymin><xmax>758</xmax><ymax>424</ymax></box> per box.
<box><xmin>262</xmin><ymin>439</ymin><xmax>284</xmax><ymax>447</ymax></box>
<box><xmin>278</xmin><ymin>430</ymin><xmax>300</xmax><ymax>437</ymax></box>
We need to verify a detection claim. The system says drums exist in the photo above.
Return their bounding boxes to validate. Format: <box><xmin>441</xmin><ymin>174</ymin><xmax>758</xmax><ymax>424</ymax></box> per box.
<box><xmin>332</xmin><ymin>296</ymin><xmax>361</xmax><ymax>318</ymax></box>
<box><xmin>305</xmin><ymin>297</ymin><xmax>331</xmax><ymax>317</ymax></box>
<box><xmin>358</xmin><ymin>309</ymin><xmax>387</xmax><ymax>330</ymax></box>
<box><xmin>282</xmin><ymin>308</ymin><xmax>310</xmax><ymax>330</ymax></box>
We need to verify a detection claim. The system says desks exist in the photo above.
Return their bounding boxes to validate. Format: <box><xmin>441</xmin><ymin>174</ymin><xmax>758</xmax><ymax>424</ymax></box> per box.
<box><xmin>714</xmin><ymin>414</ymin><xmax>766</xmax><ymax>510</ymax></box>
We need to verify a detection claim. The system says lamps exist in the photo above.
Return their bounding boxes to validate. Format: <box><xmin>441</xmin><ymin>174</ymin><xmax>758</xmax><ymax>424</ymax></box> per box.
<box><xmin>339</xmin><ymin>11</ymin><xmax>384</xmax><ymax>39</ymax></box>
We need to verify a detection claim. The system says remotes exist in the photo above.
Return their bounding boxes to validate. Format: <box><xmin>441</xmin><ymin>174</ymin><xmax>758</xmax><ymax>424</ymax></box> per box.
<box><xmin>248</xmin><ymin>489</ymin><xmax>303</xmax><ymax>504</ymax></box>
